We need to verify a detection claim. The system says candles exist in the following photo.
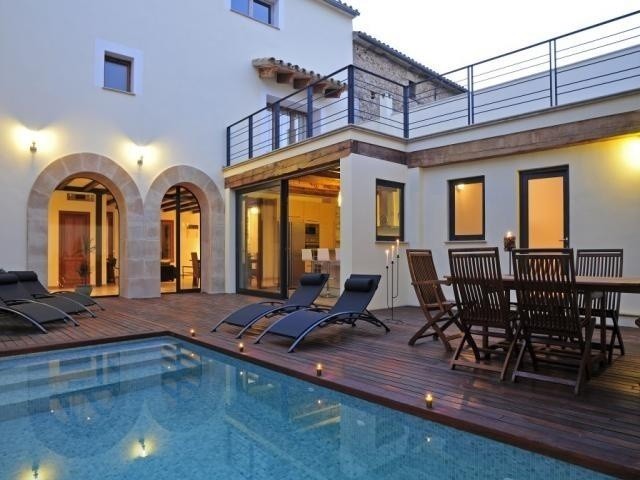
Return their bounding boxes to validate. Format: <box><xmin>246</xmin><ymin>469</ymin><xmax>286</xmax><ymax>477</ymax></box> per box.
<box><xmin>397</xmin><ymin>240</ymin><xmax>399</xmax><ymax>256</ymax></box>
<box><xmin>392</xmin><ymin>246</ymin><xmax>394</xmax><ymax>261</ymax></box>
<box><xmin>386</xmin><ymin>250</ymin><xmax>388</xmax><ymax>265</ymax></box>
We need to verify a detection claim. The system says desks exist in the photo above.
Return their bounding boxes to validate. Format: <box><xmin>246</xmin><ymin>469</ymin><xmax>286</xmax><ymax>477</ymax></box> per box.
<box><xmin>443</xmin><ymin>276</ymin><xmax>640</xmax><ymax>372</ymax></box>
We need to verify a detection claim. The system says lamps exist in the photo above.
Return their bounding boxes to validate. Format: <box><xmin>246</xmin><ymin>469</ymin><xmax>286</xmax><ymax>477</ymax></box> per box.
<box><xmin>137</xmin><ymin>155</ymin><xmax>144</xmax><ymax>166</ymax></box>
<box><xmin>29</xmin><ymin>142</ymin><xmax>37</xmax><ymax>152</ymax></box>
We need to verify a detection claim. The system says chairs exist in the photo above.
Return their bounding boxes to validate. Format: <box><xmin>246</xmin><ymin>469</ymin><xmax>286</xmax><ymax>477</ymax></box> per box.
<box><xmin>210</xmin><ymin>273</ymin><xmax>329</xmax><ymax>338</ymax></box>
<box><xmin>405</xmin><ymin>249</ymin><xmax>625</xmax><ymax>394</ymax></box>
<box><xmin>0</xmin><ymin>269</ymin><xmax>105</xmax><ymax>334</ymax></box>
<box><xmin>301</xmin><ymin>248</ymin><xmax>340</xmax><ymax>274</ymax></box>
<box><xmin>191</xmin><ymin>252</ymin><xmax>200</xmax><ymax>288</ymax></box>
<box><xmin>254</xmin><ymin>274</ymin><xmax>388</xmax><ymax>353</ymax></box>
